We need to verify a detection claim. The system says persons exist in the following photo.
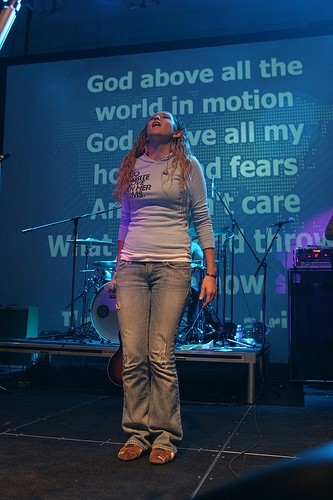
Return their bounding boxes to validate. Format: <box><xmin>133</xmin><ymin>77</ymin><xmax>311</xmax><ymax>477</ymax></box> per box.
<box><xmin>110</xmin><ymin>111</ymin><xmax>217</xmax><ymax>464</ymax></box>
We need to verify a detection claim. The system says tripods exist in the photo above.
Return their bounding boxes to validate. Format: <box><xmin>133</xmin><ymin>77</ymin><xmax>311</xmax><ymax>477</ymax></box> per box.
<box><xmin>255</xmin><ymin>225</ymin><xmax>287</xmax><ymax>397</ymax></box>
<box><xmin>197</xmin><ymin>232</ymin><xmax>255</xmax><ymax>347</ymax></box>
<box><xmin>22</xmin><ymin>207</ymin><xmax>122</xmax><ymax>343</ymax></box>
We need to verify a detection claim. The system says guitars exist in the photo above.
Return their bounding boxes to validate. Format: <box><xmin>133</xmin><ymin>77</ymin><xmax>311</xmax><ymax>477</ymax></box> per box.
<box><xmin>106</xmin><ymin>328</ymin><xmax>151</xmax><ymax>388</ymax></box>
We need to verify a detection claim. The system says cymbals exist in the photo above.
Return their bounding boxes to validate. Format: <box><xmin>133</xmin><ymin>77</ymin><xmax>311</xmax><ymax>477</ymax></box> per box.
<box><xmin>65</xmin><ymin>238</ymin><xmax>116</xmax><ymax>247</ymax></box>
<box><xmin>95</xmin><ymin>261</ymin><xmax>116</xmax><ymax>267</ymax></box>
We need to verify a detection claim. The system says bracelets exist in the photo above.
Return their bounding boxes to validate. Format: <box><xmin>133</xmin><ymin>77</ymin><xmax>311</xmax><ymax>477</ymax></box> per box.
<box><xmin>206</xmin><ymin>271</ymin><xmax>217</xmax><ymax>278</ymax></box>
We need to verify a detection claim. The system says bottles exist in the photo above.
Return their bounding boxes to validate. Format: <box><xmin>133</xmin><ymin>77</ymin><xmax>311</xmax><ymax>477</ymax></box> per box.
<box><xmin>235</xmin><ymin>325</ymin><xmax>243</xmax><ymax>347</ymax></box>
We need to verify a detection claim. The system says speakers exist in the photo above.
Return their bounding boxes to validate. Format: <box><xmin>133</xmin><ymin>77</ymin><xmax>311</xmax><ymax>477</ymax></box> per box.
<box><xmin>0</xmin><ymin>306</ymin><xmax>40</xmax><ymax>366</ymax></box>
<box><xmin>288</xmin><ymin>268</ymin><xmax>333</xmax><ymax>384</ymax></box>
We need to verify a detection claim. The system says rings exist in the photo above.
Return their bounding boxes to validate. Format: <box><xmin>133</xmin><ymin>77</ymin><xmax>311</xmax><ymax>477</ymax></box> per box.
<box><xmin>213</xmin><ymin>296</ymin><xmax>216</xmax><ymax>299</ymax></box>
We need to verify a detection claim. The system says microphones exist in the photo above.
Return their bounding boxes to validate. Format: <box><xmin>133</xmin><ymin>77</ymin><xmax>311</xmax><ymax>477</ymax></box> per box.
<box><xmin>270</xmin><ymin>218</ymin><xmax>296</xmax><ymax>228</ymax></box>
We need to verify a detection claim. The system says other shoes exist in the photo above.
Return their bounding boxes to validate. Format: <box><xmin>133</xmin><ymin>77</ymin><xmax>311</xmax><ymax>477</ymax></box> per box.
<box><xmin>118</xmin><ymin>443</ymin><xmax>144</xmax><ymax>461</ymax></box>
<box><xmin>149</xmin><ymin>448</ymin><xmax>176</xmax><ymax>465</ymax></box>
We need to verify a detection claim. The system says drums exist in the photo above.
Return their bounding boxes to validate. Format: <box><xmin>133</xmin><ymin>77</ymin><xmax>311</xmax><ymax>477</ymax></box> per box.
<box><xmin>90</xmin><ymin>267</ymin><xmax>120</xmax><ymax>345</ymax></box>
